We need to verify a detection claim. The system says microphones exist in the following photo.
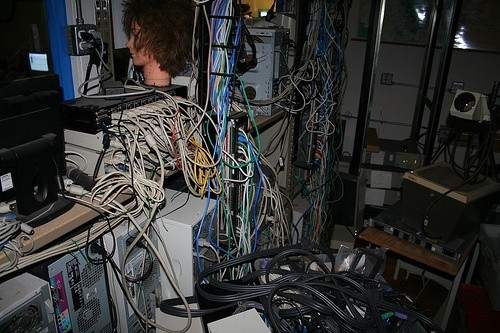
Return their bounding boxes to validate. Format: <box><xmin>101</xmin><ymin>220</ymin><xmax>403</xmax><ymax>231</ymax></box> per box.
<box><xmin>66</xmin><ymin>166</ymin><xmax>96</xmax><ymax>190</ymax></box>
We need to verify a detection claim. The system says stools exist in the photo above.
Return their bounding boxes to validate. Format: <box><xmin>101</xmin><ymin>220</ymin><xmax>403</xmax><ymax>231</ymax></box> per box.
<box><xmin>357</xmin><ymin>225</ymin><xmax>482</xmax><ymax>333</ymax></box>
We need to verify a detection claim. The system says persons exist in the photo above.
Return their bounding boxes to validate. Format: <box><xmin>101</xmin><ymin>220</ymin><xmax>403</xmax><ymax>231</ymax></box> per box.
<box><xmin>119</xmin><ymin>0</ymin><xmax>200</xmax><ymax>87</ymax></box>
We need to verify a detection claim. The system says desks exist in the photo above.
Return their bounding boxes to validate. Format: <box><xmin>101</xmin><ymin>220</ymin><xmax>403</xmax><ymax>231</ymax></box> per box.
<box><xmin>0</xmin><ymin>103</ymin><xmax>288</xmax><ymax>264</ymax></box>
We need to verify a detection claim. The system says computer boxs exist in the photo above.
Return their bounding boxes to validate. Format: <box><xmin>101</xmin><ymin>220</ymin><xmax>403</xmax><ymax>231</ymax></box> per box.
<box><xmin>106</xmin><ymin>215</ymin><xmax>159</xmax><ymax>333</ymax></box>
<box><xmin>170</xmin><ymin>161</ymin><xmax>277</xmax><ymax>263</ymax></box>
<box><xmin>0</xmin><ymin>272</ymin><xmax>58</xmax><ymax>333</ymax></box>
<box><xmin>234</xmin><ymin>25</ymin><xmax>291</xmax><ymax>115</ymax></box>
<box><xmin>30</xmin><ymin>231</ymin><xmax>113</xmax><ymax>332</ymax></box>
<box><xmin>154</xmin><ymin>187</ymin><xmax>220</xmax><ymax>333</ymax></box>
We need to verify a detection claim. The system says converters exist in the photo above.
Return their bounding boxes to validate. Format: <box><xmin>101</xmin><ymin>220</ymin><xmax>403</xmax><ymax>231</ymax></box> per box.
<box><xmin>101</xmin><ymin>80</ymin><xmax>126</xmax><ymax>99</ymax></box>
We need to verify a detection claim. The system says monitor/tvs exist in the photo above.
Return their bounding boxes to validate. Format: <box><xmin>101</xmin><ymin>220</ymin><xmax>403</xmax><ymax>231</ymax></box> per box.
<box><xmin>26</xmin><ymin>49</ymin><xmax>52</xmax><ymax>73</ymax></box>
<box><xmin>0</xmin><ymin>74</ymin><xmax>67</xmax><ymax>199</ymax></box>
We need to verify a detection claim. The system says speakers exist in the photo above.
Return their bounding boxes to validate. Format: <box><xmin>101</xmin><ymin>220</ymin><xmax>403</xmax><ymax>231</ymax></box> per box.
<box><xmin>11</xmin><ymin>150</ymin><xmax>57</xmax><ymax>214</ymax></box>
